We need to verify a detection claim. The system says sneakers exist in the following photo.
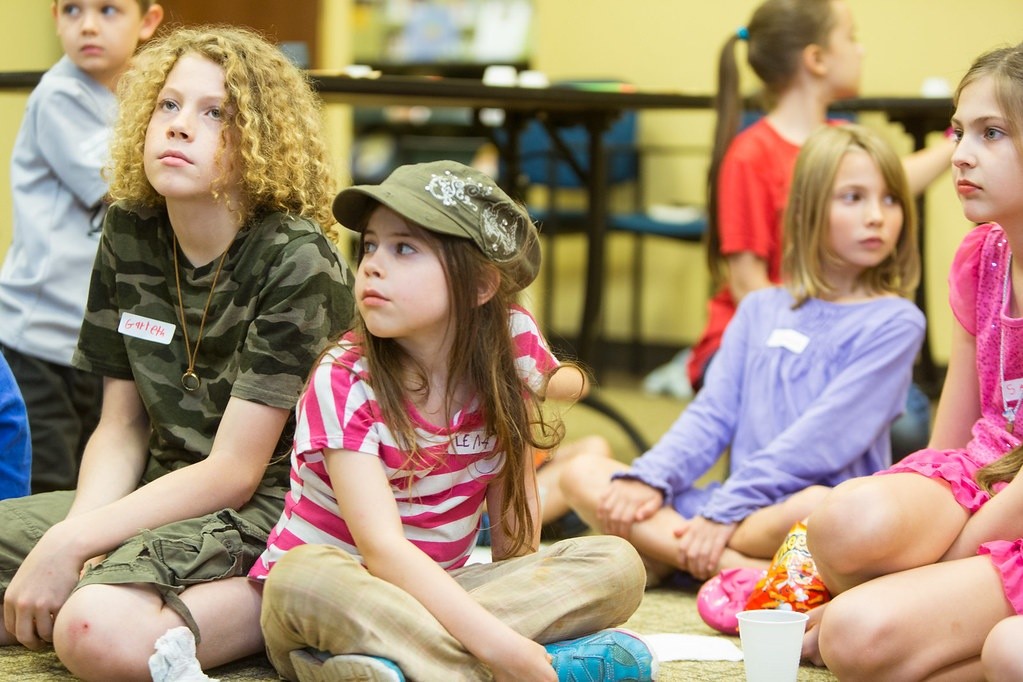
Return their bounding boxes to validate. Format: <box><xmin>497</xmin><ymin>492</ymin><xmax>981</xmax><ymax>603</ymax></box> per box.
<box><xmin>289</xmin><ymin>647</ymin><xmax>405</xmax><ymax>682</ymax></box>
<box><xmin>542</xmin><ymin>628</ymin><xmax>657</xmax><ymax>682</ymax></box>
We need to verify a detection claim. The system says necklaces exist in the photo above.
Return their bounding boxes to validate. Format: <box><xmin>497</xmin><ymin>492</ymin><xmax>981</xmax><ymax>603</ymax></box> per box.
<box><xmin>998</xmin><ymin>252</ymin><xmax>1023</xmax><ymax>435</ymax></box>
<box><xmin>174</xmin><ymin>232</ymin><xmax>234</xmax><ymax>392</ymax></box>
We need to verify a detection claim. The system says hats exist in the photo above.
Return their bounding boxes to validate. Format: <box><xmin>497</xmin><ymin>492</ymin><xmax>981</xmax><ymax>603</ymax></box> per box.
<box><xmin>333</xmin><ymin>159</ymin><xmax>541</xmax><ymax>297</ymax></box>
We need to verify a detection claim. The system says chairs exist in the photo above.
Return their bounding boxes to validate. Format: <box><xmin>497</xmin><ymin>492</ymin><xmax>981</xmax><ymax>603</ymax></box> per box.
<box><xmin>515</xmin><ymin>76</ymin><xmax>705</xmax><ymax>373</ymax></box>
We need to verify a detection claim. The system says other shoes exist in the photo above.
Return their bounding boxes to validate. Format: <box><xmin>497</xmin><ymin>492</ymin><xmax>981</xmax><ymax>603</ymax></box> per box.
<box><xmin>697</xmin><ymin>567</ymin><xmax>767</xmax><ymax>634</ymax></box>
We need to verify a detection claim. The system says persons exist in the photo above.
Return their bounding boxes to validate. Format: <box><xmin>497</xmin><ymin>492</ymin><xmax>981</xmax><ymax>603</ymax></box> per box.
<box><xmin>557</xmin><ymin>121</ymin><xmax>931</xmax><ymax>594</ymax></box>
<box><xmin>684</xmin><ymin>0</ymin><xmax>934</xmax><ymax>470</ymax></box>
<box><xmin>246</xmin><ymin>159</ymin><xmax>659</xmax><ymax>682</ymax></box>
<box><xmin>695</xmin><ymin>42</ymin><xmax>1023</xmax><ymax>682</ymax></box>
<box><xmin>477</xmin><ymin>300</ymin><xmax>607</xmax><ymax>544</ymax></box>
<box><xmin>0</xmin><ymin>0</ymin><xmax>164</xmax><ymax>493</ymax></box>
<box><xmin>0</xmin><ymin>23</ymin><xmax>357</xmax><ymax>682</ymax></box>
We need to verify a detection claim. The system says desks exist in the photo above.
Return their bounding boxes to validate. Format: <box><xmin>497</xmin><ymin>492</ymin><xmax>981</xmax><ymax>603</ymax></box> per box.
<box><xmin>735</xmin><ymin>92</ymin><xmax>961</xmax><ymax>385</ymax></box>
<box><xmin>299</xmin><ymin>68</ymin><xmax>721</xmax><ymax>382</ymax></box>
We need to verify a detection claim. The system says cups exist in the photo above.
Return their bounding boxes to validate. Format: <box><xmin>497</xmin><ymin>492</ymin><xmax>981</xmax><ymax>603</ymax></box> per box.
<box><xmin>735</xmin><ymin>609</ymin><xmax>811</xmax><ymax>682</ymax></box>
